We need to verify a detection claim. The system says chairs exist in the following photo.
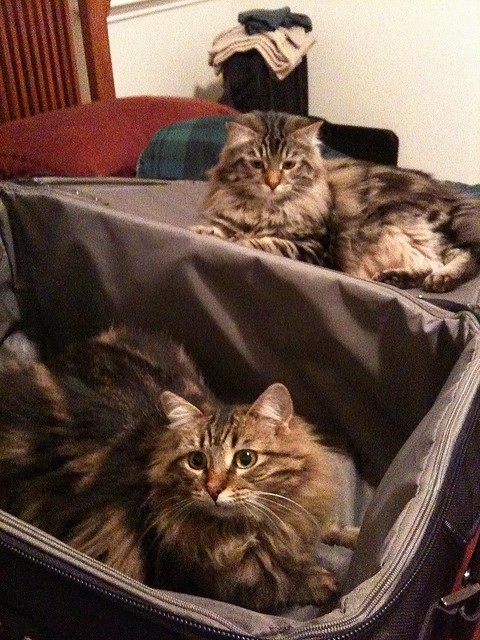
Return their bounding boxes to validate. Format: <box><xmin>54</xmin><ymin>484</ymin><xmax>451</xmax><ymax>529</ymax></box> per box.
<box><xmin>224</xmin><ymin>50</ymin><xmax>396</xmax><ymax>168</ymax></box>
<box><xmin>0</xmin><ymin>0</ymin><xmax>238</xmax><ymax>178</ymax></box>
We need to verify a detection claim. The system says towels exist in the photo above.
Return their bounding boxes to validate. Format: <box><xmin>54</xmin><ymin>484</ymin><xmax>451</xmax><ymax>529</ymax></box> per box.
<box><xmin>239</xmin><ymin>7</ymin><xmax>312</xmax><ymax>35</ymax></box>
<box><xmin>207</xmin><ymin>23</ymin><xmax>319</xmax><ymax>80</ymax></box>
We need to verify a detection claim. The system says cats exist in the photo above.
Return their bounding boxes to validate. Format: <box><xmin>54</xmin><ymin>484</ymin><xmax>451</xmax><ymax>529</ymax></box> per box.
<box><xmin>1</xmin><ymin>324</ymin><xmax>360</xmax><ymax>616</ymax></box>
<box><xmin>187</xmin><ymin>110</ymin><xmax>480</xmax><ymax>294</ymax></box>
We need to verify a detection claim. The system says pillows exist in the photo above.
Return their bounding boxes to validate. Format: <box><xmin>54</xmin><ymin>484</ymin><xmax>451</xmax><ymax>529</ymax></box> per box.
<box><xmin>0</xmin><ymin>95</ymin><xmax>243</xmax><ymax>179</ymax></box>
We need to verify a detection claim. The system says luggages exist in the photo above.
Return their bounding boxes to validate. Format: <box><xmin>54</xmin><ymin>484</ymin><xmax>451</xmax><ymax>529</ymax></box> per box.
<box><xmin>0</xmin><ymin>145</ymin><xmax>480</xmax><ymax>640</ymax></box>
